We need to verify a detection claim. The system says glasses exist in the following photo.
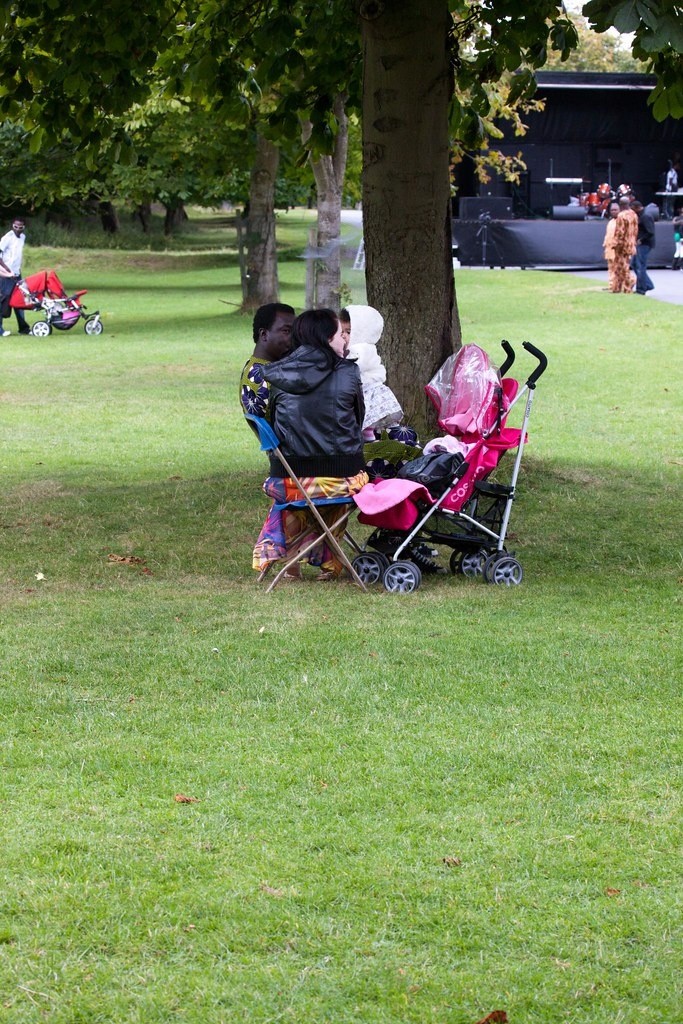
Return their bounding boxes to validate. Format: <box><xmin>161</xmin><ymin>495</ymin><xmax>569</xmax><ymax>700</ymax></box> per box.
<box><xmin>12</xmin><ymin>223</ymin><xmax>26</xmax><ymax>231</ymax></box>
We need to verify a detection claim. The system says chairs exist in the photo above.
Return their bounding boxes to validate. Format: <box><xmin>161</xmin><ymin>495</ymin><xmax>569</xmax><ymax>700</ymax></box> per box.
<box><xmin>242</xmin><ymin>412</ymin><xmax>373</xmax><ymax>594</ymax></box>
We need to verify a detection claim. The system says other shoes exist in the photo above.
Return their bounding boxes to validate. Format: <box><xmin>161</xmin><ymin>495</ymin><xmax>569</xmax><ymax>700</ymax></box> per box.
<box><xmin>19</xmin><ymin>329</ymin><xmax>33</xmax><ymax>335</ymax></box>
<box><xmin>2</xmin><ymin>330</ymin><xmax>11</xmax><ymax>337</ymax></box>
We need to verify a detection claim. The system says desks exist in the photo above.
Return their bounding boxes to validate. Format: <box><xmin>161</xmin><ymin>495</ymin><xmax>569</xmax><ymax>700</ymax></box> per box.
<box><xmin>453</xmin><ymin>218</ymin><xmax>676</xmax><ymax>270</ymax></box>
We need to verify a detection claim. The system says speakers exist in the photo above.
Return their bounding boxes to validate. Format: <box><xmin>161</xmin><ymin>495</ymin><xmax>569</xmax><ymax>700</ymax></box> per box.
<box><xmin>459</xmin><ymin>196</ymin><xmax>512</xmax><ymax>220</ymax></box>
<box><xmin>645</xmin><ymin>206</ymin><xmax>659</xmax><ymax>221</ymax></box>
<box><xmin>552</xmin><ymin>205</ymin><xmax>586</xmax><ymax>221</ymax></box>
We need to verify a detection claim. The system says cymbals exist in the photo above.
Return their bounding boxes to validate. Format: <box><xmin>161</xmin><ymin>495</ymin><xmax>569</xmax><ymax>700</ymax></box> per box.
<box><xmin>582</xmin><ymin>181</ymin><xmax>591</xmax><ymax>183</ymax></box>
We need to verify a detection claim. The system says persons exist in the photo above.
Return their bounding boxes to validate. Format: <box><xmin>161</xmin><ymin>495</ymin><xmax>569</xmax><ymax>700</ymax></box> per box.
<box><xmin>672</xmin><ymin>208</ymin><xmax>683</xmax><ymax>271</ymax></box>
<box><xmin>239</xmin><ymin>303</ymin><xmax>405</xmax><ymax>580</ymax></box>
<box><xmin>0</xmin><ymin>216</ymin><xmax>33</xmax><ymax>336</ymax></box>
<box><xmin>664</xmin><ymin>161</ymin><xmax>680</xmax><ymax>220</ymax></box>
<box><xmin>579</xmin><ymin>183</ymin><xmax>655</xmax><ymax>295</ymax></box>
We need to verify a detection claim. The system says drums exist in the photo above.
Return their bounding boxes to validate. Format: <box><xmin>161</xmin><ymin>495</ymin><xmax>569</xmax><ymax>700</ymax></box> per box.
<box><xmin>588</xmin><ymin>193</ymin><xmax>601</xmax><ymax>207</ymax></box>
<box><xmin>616</xmin><ymin>184</ymin><xmax>631</xmax><ymax>196</ymax></box>
<box><xmin>577</xmin><ymin>193</ymin><xmax>590</xmax><ymax>206</ymax></box>
<box><xmin>618</xmin><ymin>194</ymin><xmax>635</xmax><ymax>203</ymax></box>
<box><xmin>597</xmin><ymin>183</ymin><xmax>611</xmax><ymax>198</ymax></box>
<box><xmin>599</xmin><ymin>197</ymin><xmax>610</xmax><ymax>218</ymax></box>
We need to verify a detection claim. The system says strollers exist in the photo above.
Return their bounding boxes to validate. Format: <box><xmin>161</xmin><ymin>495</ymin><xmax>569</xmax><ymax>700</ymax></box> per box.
<box><xmin>9</xmin><ymin>271</ymin><xmax>104</xmax><ymax>338</ymax></box>
<box><xmin>353</xmin><ymin>340</ymin><xmax>548</xmax><ymax>597</ymax></box>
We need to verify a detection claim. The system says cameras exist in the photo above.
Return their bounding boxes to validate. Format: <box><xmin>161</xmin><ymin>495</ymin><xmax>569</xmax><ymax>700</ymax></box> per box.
<box><xmin>479</xmin><ymin>212</ymin><xmax>490</xmax><ymax>222</ymax></box>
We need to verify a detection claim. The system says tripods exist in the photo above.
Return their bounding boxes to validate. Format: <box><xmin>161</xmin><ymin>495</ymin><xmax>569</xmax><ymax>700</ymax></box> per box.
<box><xmin>466</xmin><ymin>222</ymin><xmax>505</xmax><ymax>269</ymax></box>
